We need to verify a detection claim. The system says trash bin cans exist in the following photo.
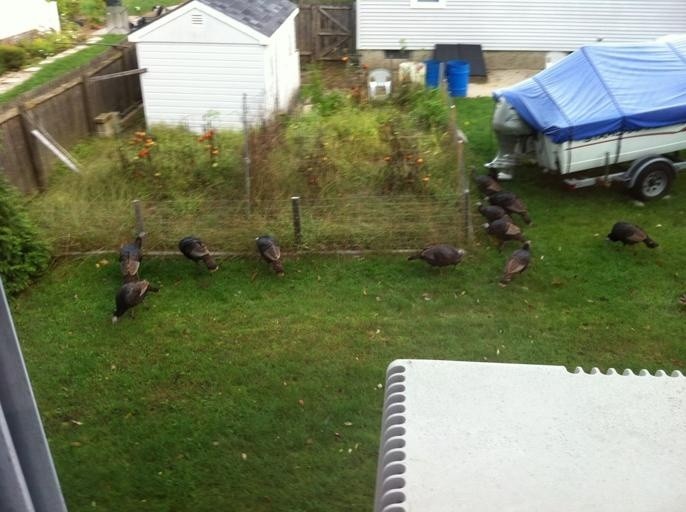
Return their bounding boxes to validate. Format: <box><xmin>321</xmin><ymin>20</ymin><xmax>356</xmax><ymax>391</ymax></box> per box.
<box><xmin>445</xmin><ymin>60</ymin><xmax>471</xmax><ymax>97</ymax></box>
<box><xmin>423</xmin><ymin>60</ymin><xmax>440</xmax><ymax>88</ymax></box>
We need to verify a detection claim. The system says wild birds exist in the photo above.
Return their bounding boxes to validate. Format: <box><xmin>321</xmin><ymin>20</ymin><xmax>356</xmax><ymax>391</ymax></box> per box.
<box><xmin>605</xmin><ymin>221</ymin><xmax>659</xmax><ymax>252</ymax></box>
<box><xmin>255</xmin><ymin>235</ymin><xmax>285</xmax><ymax>277</ymax></box>
<box><xmin>467</xmin><ymin>165</ymin><xmax>534</xmax><ymax>247</ymax></box>
<box><xmin>111</xmin><ymin>279</ymin><xmax>160</xmax><ymax>326</ymax></box>
<box><xmin>498</xmin><ymin>240</ymin><xmax>532</xmax><ymax>288</ymax></box>
<box><xmin>407</xmin><ymin>243</ymin><xmax>466</xmax><ymax>276</ymax></box>
<box><xmin>118</xmin><ymin>236</ymin><xmax>144</xmax><ymax>284</ymax></box>
<box><xmin>178</xmin><ymin>237</ymin><xmax>219</xmax><ymax>272</ymax></box>
